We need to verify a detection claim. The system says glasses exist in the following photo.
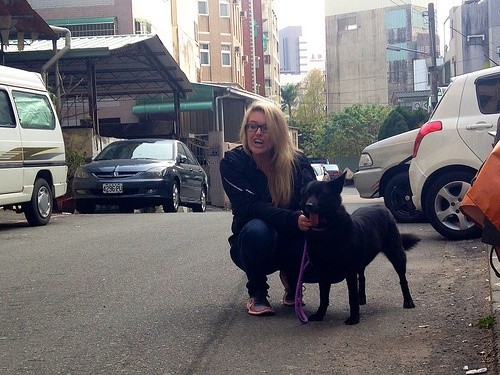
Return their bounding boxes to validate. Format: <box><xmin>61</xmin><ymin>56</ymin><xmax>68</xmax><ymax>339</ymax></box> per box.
<box><xmin>245</xmin><ymin>123</ymin><xmax>270</xmax><ymax>134</ymax></box>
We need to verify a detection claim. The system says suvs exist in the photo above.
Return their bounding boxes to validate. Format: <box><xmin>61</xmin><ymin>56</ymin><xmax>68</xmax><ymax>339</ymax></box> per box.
<box><xmin>408</xmin><ymin>65</ymin><xmax>500</xmax><ymax>241</ymax></box>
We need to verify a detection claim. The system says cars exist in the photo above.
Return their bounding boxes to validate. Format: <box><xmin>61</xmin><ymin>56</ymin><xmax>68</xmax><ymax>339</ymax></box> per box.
<box><xmin>352</xmin><ymin>127</ymin><xmax>426</xmax><ymax>223</ymax></box>
<box><xmin>71</xmin><ymin>138</ymin><xmax>209</xmax><ymax>213</ymax></box>
<box><xmin>323</xmin><ymin>163</ymin><xmax>340</xmax><ymax>180</ymax></box>
<box><xmin>310</xmin><ymin>163</ymin><xmax>331</xmax><ymax>181</ymax></box>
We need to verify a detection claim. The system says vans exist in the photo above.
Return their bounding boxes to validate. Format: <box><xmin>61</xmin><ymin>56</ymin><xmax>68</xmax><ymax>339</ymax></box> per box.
<box><xmin>0</xmin><ymin>62</ymin><xmax>68</xmax><ymax>227</ymax></box>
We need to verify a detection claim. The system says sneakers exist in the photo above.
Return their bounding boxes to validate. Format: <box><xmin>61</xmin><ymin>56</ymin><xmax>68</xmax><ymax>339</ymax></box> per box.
<box><xmin>247</xmin><ymin>293</ymin><xmax>275</xmax><ymax>315</ymax></box>
<box><xmin>283</xmin><ymin>284</ymin><xmax>308</xmax><ymax>306</ymax></box>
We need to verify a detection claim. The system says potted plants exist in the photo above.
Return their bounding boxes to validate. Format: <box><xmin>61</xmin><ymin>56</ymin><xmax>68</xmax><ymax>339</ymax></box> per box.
<box><xmin>55</xmin><ymin>147</ymin><xmax>88</xmax><ymax>212</ymax></box>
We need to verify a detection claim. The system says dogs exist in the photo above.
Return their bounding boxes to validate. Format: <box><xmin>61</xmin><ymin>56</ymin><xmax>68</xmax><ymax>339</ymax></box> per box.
<box><xmin>296</xmin><ymin>170</ymin><xmax>420</xmax><ymax>326</ymax></box>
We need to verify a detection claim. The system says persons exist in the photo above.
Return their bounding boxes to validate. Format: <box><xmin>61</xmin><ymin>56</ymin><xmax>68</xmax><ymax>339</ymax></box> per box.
<box><xmin>219</xmin><ymin>99</ymin><xmax>318</xmax><ymax>314</ymax></box>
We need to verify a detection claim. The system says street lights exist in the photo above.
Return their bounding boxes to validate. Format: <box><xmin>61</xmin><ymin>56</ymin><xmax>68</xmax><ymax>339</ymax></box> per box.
<box><xmin>384</xmin><ymin>44</ymin><xmax>439</xmax><ymax>109</ymax></box>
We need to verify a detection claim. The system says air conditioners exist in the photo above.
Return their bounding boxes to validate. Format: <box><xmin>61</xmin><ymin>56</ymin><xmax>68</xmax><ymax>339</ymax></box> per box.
<box><xmin>240</xmin><ymin>11</ymin><xmax>248</xmax><ymax>19</ymax></box>
<box><xmin>242</xmin><ymin>55</ymin><xmax>248</xmax><ymax>62</ymax></box>
